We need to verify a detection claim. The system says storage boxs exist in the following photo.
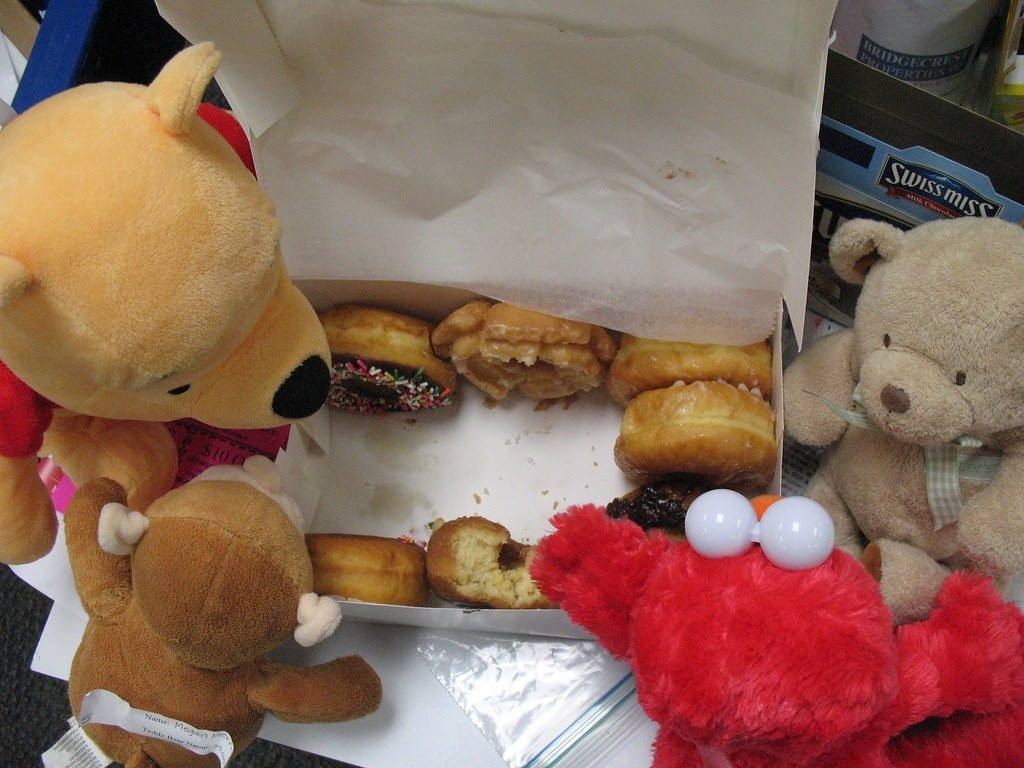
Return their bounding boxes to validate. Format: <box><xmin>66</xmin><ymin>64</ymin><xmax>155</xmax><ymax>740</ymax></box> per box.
<box><xmin>155</xmin><ymin>0</ymin><xmax>1024</xmax><ymax>640</ymax></box>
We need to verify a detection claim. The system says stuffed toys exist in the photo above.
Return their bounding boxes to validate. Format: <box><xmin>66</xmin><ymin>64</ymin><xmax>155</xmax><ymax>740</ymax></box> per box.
<box><xmin>63</xmin><ymin>454</ymin><xmax>383</xmax><ymax>768</ymax></box>
<box><xmin>0</xmin><ymin>43</ymin><xmax>332</xmax><ymax>566</ymax></box>
<box><xmin>527</xmin><ymin>489</ymin><xmax>1024</xmax><ymax>768</ymax></box>
<box><xmin>782</xmin><ymin>217</ymin><xmax>1024</xmax><ymax>626</ymax></box>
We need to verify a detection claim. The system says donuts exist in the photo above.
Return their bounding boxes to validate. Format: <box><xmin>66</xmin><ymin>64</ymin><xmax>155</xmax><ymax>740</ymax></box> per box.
<box><xmin>301</xmin><ymin>299</ymin><xmax>776</xmax><ymax>611</ymax></box>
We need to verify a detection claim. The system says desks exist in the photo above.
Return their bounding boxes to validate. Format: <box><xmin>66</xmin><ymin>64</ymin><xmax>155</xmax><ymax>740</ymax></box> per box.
<box><xmin>32</xmin><ymin>565</ymin><xmax>658</xmax><ymax>768</ymax></box>
